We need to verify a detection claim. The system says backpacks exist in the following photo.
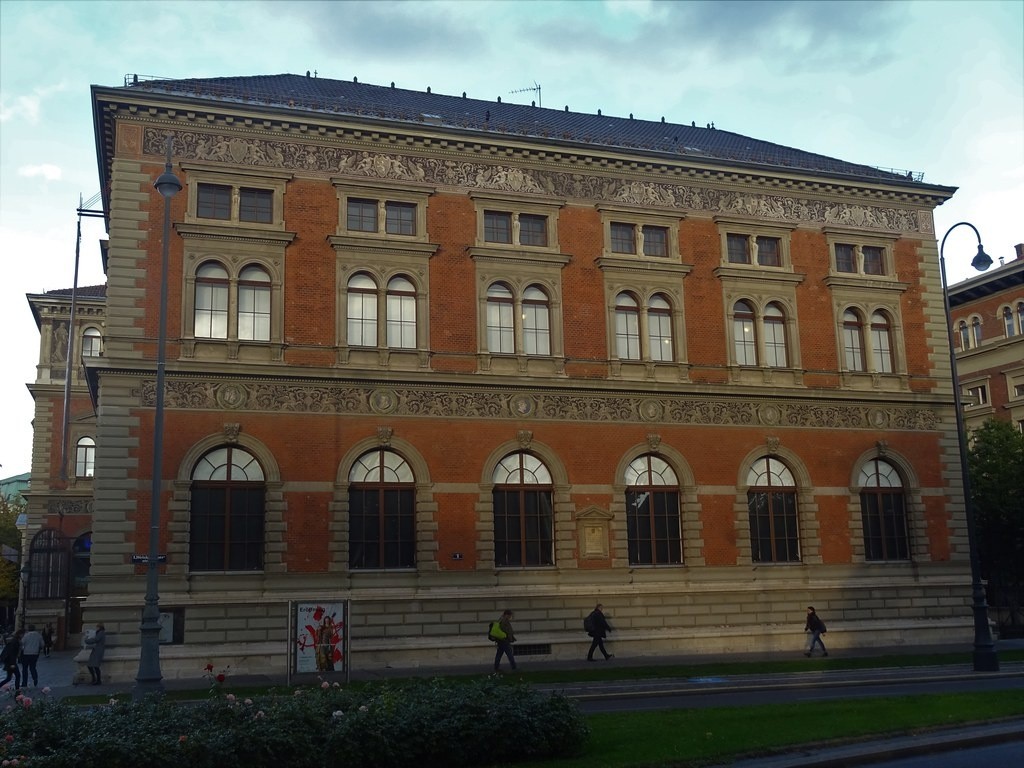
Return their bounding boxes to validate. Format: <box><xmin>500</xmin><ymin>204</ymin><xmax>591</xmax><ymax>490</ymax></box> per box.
<box><xmin>488</xmin><ymin>617</ymin><xmax>507</xmax><ymax>642</ymax></box>
<box><xmin>584</xmin><ymin>611</ymin><xmax>595</xmax><ymax>632</ymax></box>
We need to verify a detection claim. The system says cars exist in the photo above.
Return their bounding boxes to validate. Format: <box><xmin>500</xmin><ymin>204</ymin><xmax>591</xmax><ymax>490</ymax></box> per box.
<box><xmin>3</xmin><ymin>632</ymin><xmax>15</xmax><ymax>644</ymax></box>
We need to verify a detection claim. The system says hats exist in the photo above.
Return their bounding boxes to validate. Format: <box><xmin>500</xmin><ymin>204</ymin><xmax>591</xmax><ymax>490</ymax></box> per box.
<box><xmin>503</xmin><ymin>610</ymin><xmax>513</xmax><ymax>615</ymax></box>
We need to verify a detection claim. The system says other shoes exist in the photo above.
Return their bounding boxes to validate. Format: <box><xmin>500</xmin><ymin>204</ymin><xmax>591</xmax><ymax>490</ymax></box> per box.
<box><xmin>821</xmin><ymin>652</ymin><xmax>828</xmax><ymax>657</ymax></box>
<box><xmin>513</xmin><ymin>666</ymin><xmax>520</xmax><ymax>671</ymax></box>
<box><xmin>804</xmin><ymin>652</ymin><xmax>811</xmax><ymax>657</ymax></box>
<box><xmin>606</xmin><ymin>654</ymin><xmax>612</xmax><ymax>660</ymax></box>
<box><xmin>91</xmin><ymin>680</ymin><xmax>102</xmax><ymax>685</ymax></box>
<box><xmin>587</xmin><ymin>657</ymin><xmax>596</xmax><ymax>662</ymax></box>
<box><xmin>495</xmin><ymin>667</ymin><xmax>504</xmax><ymax>671</ymax></box>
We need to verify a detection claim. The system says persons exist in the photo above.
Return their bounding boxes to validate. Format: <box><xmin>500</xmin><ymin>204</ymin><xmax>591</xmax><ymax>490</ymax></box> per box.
<box><xmin>315</xmin><ymin>616</ymin><xmax>335</xmax><ymax>672</ymax></box>
<box><xmin>804</xmin><ymin>606</ymin><xmax>828</xmax><ymax>658</ymax></box>
<box><xmin>494</xmin><ymin>609</ymin><xmax>520</xmax><ymax>672</ymax></box>
<box><xmin>586</xmin><ymin>604</ymin><xmax>612</xmax><ymax>662</ymax></box>
<box><xmin>83</xmin><ymin>622</ymin><xmax>106</xmax><ymax>686</ymax></box>
<box><xmin>18</xmin><ymin>625</ymin><xmax>45</xmax><ymax>687</ymax></box>
<box><xmin>42</xmin><ymin>622</ymin><xmax>55</xmax><ymax>658</ymax></box>
<box><xmin>0</xmin><ymin>630</ymin><xmax>25</xmax><ymax>690</ymax></box>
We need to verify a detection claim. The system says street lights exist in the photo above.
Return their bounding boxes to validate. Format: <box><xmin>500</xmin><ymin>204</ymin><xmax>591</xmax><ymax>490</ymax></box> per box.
<box><xmin>136</xmin><ymin>134</ymin><xmax>183</xmax><ymax>697</ymax></box>
<box><xmin>941</xmin><ymin>224</ymin><xmax>999</xmax><ymax>672</ymax></box>
